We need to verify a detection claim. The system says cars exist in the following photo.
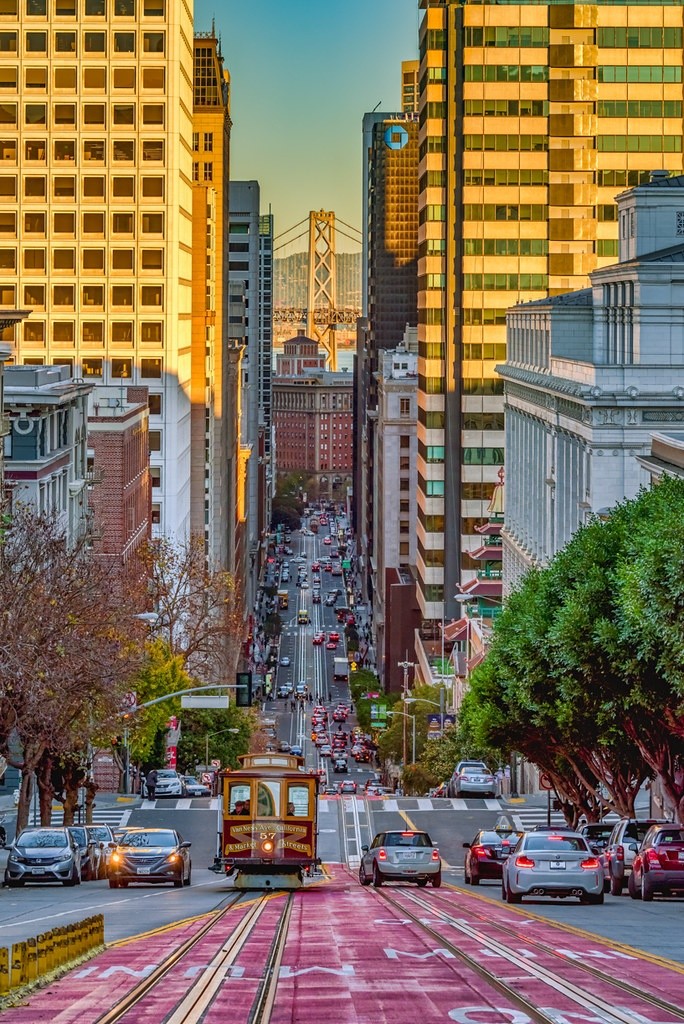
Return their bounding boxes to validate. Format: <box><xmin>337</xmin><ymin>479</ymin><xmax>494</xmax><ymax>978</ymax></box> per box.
<box><xmin>423</xmin><ymin>761</ymin><xmax>496</xmax><ymax>800</ymax></box>
<box><xmin>262</xmin><ymin>680</ymin><xmax>392</xmax><ymax>796</ymax></box>
<box><xmin>462</xmin><ymin>820</ymin><xmax>684</xmax><ymax>903</ymax></box>
<box><xmin>141</xmin><ymin>769</ymin><xmax>212</xmax><ymax>799</ymax></box>
<box><xmin>269</xmin><ymin>503</ymin><xmax>356</xmax><ymax>626</ymax></box>
<box><xmin>280</xmin><ymin>657</ymin><xmax>290</xmax><ymax>666</ymax></box>
<box><xmin>312</xmin><ymin>630</ymin><xmax>340</xmax><ymax>649</ymax></box>
<box><xmin>4</xmin><ymin>823</ymin><xmax>116</xmax><ymax>887</ymax></box>
<box><xmin>359</xmin><ymin>830</ymin><xmax>442</xmax><ymax>888</ymax></box>
<box><xmin>107</xmin><ymin>827</ymin><xmax>193</xmax><ymax>887</ymax></box>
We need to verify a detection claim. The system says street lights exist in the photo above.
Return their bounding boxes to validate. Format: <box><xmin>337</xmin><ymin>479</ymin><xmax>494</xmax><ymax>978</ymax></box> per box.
<box><xmin>205</xmin><ymin>728</ymin><xmax>240</xmax><ymax>770</ymax></box>
<box><xmin>86</xmin><ymin>613</ymin><xmax>160</xmax><ymax>788</ymax></box>
<box><xmin>385</xmin><ymin>710</ymin><xmax>416</xmax><ymax>766</ymax></box>
<box><xmin>404</xmin><ymin>689</ymin><xmax>446</xmax><ymax>747</ymax></box>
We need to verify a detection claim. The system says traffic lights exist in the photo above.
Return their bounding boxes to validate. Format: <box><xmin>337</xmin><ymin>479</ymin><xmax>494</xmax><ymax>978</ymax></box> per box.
<box><xmin>111</xmin><ymin>736</ymin><xmax>122</xmax><ymax>745</ymax></box>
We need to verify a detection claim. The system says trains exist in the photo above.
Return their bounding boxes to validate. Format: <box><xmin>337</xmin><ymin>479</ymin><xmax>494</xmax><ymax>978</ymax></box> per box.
<box><xmin>209</xmin><ymin>753</ymin><xmax>323</xmax><ymax>889</ymax></box>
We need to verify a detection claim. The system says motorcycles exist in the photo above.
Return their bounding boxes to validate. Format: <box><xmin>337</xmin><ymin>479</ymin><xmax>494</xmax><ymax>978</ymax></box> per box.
<box><xmin>0</xmin><ymin>826</ymin><xmax>7</xmax><ymax>846</ymax></box>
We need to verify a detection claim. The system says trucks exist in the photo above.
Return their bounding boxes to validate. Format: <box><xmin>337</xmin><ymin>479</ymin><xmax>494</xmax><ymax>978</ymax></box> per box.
<box><xmin>333</xmin><ymin>657</ymin><xmax>348</xmax><ymax>680</ymax></box>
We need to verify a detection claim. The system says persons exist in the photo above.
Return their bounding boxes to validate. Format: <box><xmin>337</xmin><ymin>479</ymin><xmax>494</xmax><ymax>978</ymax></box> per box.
<box><xmin>244</xmin><ymin>799</ymin><xmax>251</xmax><ymax>811</ymax></box>
<box><xmin>250</xmin><ymin>590</ymin><xmax>275</xmax><ymax>654</ymax></box>
<box><xmin>338</xmin><ymin>723</ymin><xmax>342</xmax><ymax>731</ymax></box>
<box><xmin>266</xmin><ymin>687</ymin><xmax>354</xmax><ymax>714</ymax></box>
<box><xmin>287</xmin><ymin>802</ymin><xmax>295</xmax><ymax>816</ymax></box>
<box><xmin>229</xmin><ymin>801</ymin><xmax>250</xmax><ymax>816</ymax></box>
<box><xmin>344</xmin><ymin>554</ymin><xmax>375</xmax><ymax>673</ymax></box>
<box><xmin>145</xmin><ymin>771</ymin><xmax>158</xmax><ymax>801</ymax></box>
